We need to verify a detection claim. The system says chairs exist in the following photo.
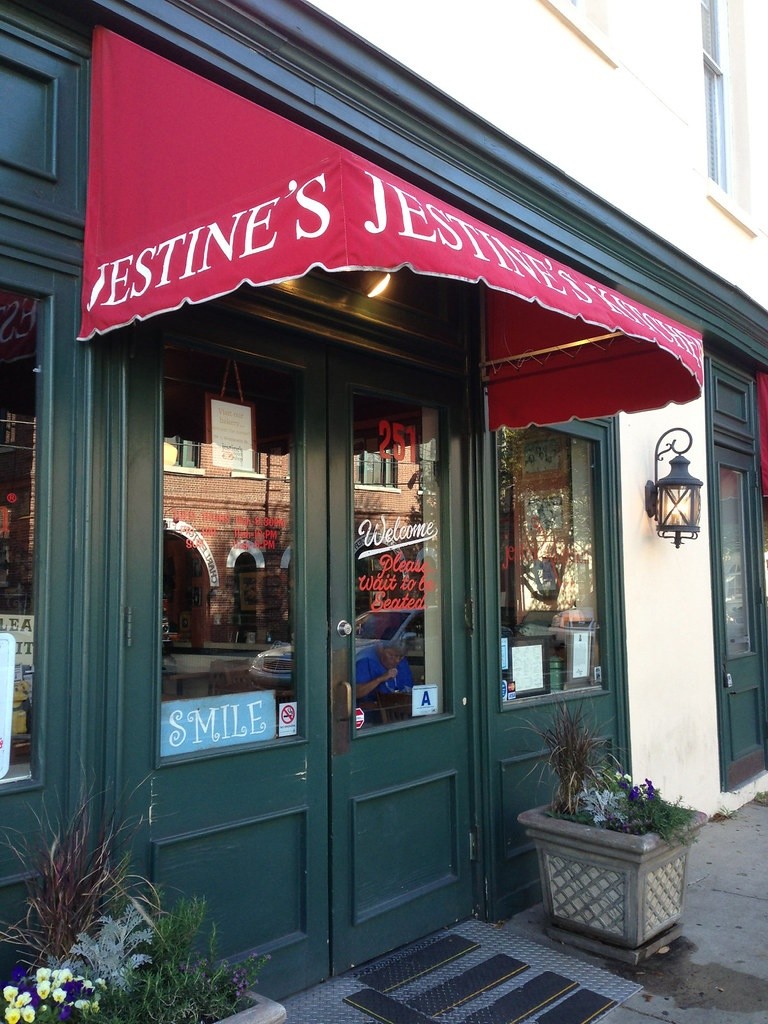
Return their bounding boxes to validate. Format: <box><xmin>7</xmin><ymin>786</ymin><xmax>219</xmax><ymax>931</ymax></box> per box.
<box><xmin>374</xmin><ymin>693</ymin><xmax>412</xmax><ymax>724</ymax></box>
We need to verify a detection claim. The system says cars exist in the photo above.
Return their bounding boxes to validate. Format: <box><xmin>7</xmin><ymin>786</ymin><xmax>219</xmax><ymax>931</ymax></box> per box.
<box><xmin>247</xmin><ymin>605</ymin><xmax>424</xmax><ymax>697</ymax></box>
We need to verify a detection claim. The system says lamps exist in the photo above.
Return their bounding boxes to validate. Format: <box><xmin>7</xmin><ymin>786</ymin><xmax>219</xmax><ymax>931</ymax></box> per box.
<box><xmin>645</xmin><ymin>427</ymin><xmax>704</xmax><ymax>549</ymax></box>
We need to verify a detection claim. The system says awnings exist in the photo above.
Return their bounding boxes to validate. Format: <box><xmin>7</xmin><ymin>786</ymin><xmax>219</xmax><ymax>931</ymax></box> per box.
<box><xmin>78</xmin><ymin>23</ymin><xmax>703</xmax><ymax>433</ymax></box>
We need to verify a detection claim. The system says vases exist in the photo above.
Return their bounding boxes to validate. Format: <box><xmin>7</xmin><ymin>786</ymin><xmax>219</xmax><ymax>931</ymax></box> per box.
<box><xmin>516</xmin><ymin>802</ymin><xmax>709</xmax><ymax>967</ymax></box>
<box><xmin>204</xmin><ymin>987</ymin><xmax>288</xmax><ymax>1024</ymax></box>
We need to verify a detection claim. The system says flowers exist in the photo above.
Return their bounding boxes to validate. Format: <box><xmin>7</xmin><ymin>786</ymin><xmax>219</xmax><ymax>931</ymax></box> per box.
<box><xmin>503</xmin><ymin>682</ymin><xmax>700</xmax><ymax>852</ymax></box>
<box><xmin>0</xmin><ymin>755</ymin><xmax>274</xmax><ymax>1024</ymax></box>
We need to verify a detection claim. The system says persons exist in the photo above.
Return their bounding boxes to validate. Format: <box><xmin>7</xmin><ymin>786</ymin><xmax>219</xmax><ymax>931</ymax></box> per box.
<box><xmin>355</xmin><ymin>626</ymin><xmax>413</xmax><ymax>731</ymax></box>
<box><xmin>271</xmin><ymin>610</ymin><xmax>291</xmax><ymax>644</ymax></box>
